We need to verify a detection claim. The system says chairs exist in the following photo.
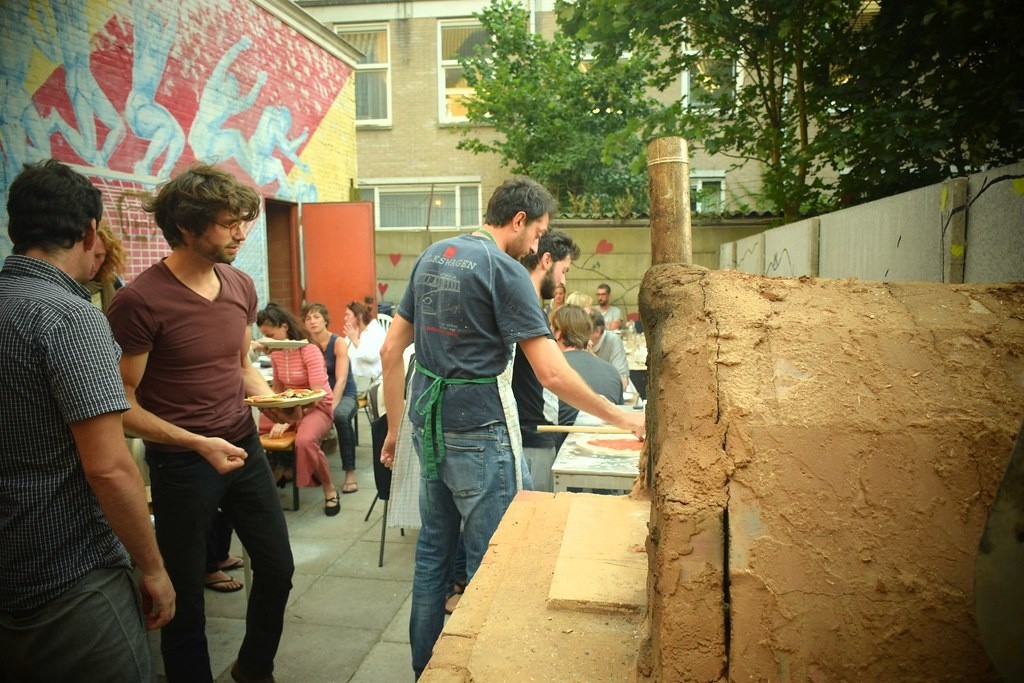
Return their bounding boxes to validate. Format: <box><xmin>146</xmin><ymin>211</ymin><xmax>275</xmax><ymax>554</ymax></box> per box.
<box><xmin>352</xmin><ymin>374</ymin><xmax>404</xmax><ymax>568</ymax></box>
<box><xmin>259</xmin><ymin>429</ymin><xmax>301</xmax><ymax>511</ymax></box>
<box><xmin>377</xmin><ymin>313</ymin><xmax>393</xmax><ymax>333</ymax></box>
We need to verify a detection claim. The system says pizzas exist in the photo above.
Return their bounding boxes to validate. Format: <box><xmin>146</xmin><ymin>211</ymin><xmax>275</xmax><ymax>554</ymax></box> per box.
<box><xmin>249</xmin><ymin>389</ymin><xmax>323</xmax><ymax>402</ymax></box>
<box><xmin>575</xmin><ymin>434</ymin><xmax>646</xmax><ymax>456</ymax></box>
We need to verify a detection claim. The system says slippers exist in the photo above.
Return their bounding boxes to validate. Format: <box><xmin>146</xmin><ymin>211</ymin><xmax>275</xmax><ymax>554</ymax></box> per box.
<box><xmin>208</xmin><ymin>556</ymin><xmax>245</xmax><ymax>572</ymax></box>
<box><xmin>342</xmin><ymin>475</ymin><xmax>358</xmax><ymax>494</ymax></box>
<box><xmin>203</xmin><ymin>576</ymin><xmax>243</xmax><ymax>593</ymax></box>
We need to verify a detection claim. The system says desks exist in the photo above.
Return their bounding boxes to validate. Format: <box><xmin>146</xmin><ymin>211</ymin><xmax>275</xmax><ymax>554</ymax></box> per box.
<box><xmin>550</xmin><ymin>405</ymin><xmax>646</xmax><ymax>493</ymax></box>
<box><xmin>623</xmin><ymin>335</ymin><xmax>648</xmax><ymax>401</ymax></box>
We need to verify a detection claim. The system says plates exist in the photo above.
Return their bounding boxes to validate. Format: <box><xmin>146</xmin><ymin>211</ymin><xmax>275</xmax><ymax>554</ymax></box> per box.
<box><xmin>258</xmin><ymin>340</ymin><xmax>309</xmax><ymax>349</ymax></box>
<box><xmin>245</xmin><ymin>390</ymin><xmax>327</xmax><ymax>408</ymax></box>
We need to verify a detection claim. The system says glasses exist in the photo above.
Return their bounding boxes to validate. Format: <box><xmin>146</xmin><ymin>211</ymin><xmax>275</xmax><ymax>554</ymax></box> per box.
<box><xmin>204</xmin><ymin>217</ymin><xmax>242</xmax><ymax>230</ymax></box>
<box><xmin>596</xmin><ymin>292</ymin><xmax>608</xmax><ymax>296</ymax></box>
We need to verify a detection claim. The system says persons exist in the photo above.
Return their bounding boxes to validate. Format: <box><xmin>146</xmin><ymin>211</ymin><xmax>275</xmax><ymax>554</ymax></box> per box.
<box><xmin>77</xmin><ymin>225</ymin><xmax>123</xmax><ymax>309</ymax></box>
<box><xmin>105</xmin><ymin>168</ymin><xmax>295</xmax><ymax>683</ymax></box>
<box><xmin>0</xmin><ymin>161</ymin><xmax>177</xmax><ymax>683</ymax></box>
<box><xmin>376</xmin><ymin>177</ymin><xmax>648</xmax><ymax>682</ymax></box>
<box><xmin>122</xmin><ymin>282</ymin><xmax>642</xmax><ymax>616</ymax></box>
<box><xmin>512</xmin><ymin>227</ymin><xmax>581</xmax><ymax>493</ymax></box>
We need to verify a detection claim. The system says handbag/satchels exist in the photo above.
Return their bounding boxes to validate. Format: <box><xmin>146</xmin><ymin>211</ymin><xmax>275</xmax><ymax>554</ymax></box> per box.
<box><xmin>352</xmin><ymin>373</ymin><xmax>373</xmax><ymax>400</ymax></box>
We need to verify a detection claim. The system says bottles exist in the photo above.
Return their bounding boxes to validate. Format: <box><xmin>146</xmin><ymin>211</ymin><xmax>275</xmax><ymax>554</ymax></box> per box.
<box><xmin>626</xmin><ymin>319</ymin><xmax>636</xmax><ymax>349</ymax></box>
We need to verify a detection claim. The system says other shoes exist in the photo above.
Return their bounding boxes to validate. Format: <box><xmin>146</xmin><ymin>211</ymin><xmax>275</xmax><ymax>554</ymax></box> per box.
<box><xmin>230</xmin><ymin>659</ymin><xmax>276</xmax><ymax>683</ymax></box>
<box><xmin>282</xmin><ymin>466</ymin><xmax>293</xmax><ymax>483</ymax></box>
<box><xmin>444</xmin><ymin>582</ymin><xmax>465</xmax><ymax>614</ymax></box>
<box><xmin>324</xmin><ymin>489</ymin><xmax>340</xmax><ymax>516</ymax></box>
